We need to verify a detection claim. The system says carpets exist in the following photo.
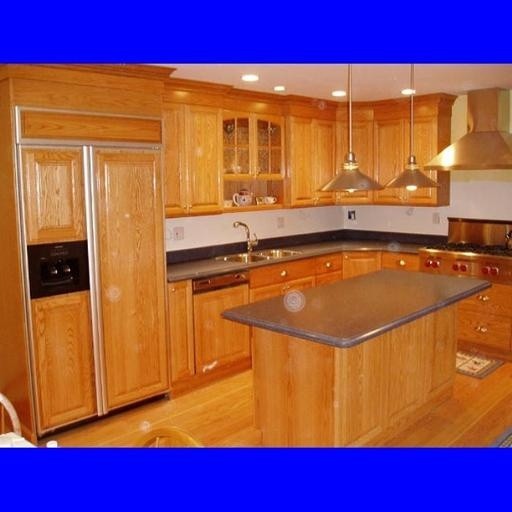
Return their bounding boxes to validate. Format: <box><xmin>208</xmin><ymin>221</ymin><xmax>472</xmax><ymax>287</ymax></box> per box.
<box><xmin>455</xmin><ymin>351</ymin><xmax>503</xmax><ymax>377</ymax></box>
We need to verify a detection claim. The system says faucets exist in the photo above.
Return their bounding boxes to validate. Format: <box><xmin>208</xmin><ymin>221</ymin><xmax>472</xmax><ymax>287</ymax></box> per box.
<box><xmin>232</xmin><ymin>221</ymin><xmax>257</xmax><ymax>254</ymax></box>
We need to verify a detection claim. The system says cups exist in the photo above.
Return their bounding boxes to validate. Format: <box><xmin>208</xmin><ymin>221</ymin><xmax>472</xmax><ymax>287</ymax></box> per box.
<box><xmin>265</xmin><ymin>196</ymin><xmax>277</xmax><ymax>205</ymax></box>
<box><xmin>224</xmin><ymin>200</ymin><xmax>233</xmax><ymax>208</ymax></box>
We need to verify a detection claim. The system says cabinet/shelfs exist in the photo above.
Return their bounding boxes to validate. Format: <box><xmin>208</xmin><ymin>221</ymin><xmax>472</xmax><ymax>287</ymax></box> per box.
<box><xmin>162</xmin><ymin>80</ymin><xmax>225</xmax><ymax>216</ymax></box>
<box><xmin>249</xmin><ymin>260</ymin><xmax>318</xmax><ymax>301</ymax></box>
<box><xmin>381</xmin><ymin>250</ymin><xmax>421</xmax><ymax>270</ymax></box>
<box><xmin>1</xmin><ymin>143</ymin><xmax>86</xmax><ymax>247</ymax></box>
<box><xmin>373</xmin><ymin>92</ymin><xmax>454</xmax><ymax>208</ymax></box>
<box><xmin>167</xmin><ymin>275</ymin><xmax>250</xmax><ymax>401</ymax></box>
<box><xmin>452</xmin><ymin>284</ymin><xmax>511</xmax><ymax>351</ymax></box>
<box><xmin>223</xmin><ymin>85</ymin><xmax>287</xmax><ymax>210</ymax></box>
<box><xmin>320</xmin><ymin>253</ymin><xmax>380</xmax><ymax>284</ymax></box>
<box><xmin>0</xmin><ymin>291</ymin><xmax>99</xmax><ymax>446</ymax></box>
<box><xmin>288</xmin><ymin>93</ymin><xmax>336</xmax><ymax>207</ymax></box>
<box><xmin>334</xmin><ymin>98</ymin><xmax>374</xmax><ymax>204</ymax></box>
<box><xmin>87</xmin><ymin>144</ymin><xmax>171</xmax><ymax>416</ymax></box>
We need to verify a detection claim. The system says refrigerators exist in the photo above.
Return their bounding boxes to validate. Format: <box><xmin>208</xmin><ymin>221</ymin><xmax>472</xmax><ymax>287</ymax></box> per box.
<box><xmin>17</xmin><ymin>106</ymin><xmax>174</xmax><ymax>438</ymax></box>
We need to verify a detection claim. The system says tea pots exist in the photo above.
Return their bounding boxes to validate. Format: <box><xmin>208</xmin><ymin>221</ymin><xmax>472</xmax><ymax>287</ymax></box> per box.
<box><xmin>505</xmin><ymin>231</ymin><xmax>512</xmax><ymax>250</ymax></box>
<box><xmin>232</xmin><ymin>189</ymin><xmax>254</xmax><ymax>208</ymax></box>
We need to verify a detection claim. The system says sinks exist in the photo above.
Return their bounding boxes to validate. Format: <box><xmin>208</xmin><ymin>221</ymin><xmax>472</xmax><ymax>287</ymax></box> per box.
<box><xmin>254</xmin><ymin>247</ymin><xmax>301</xmax><ymax>258</ymax></box>
<box><xmin>223</xmin><ymin>253</ymin><xmax>269</xmax><ymax>264</ymax></box>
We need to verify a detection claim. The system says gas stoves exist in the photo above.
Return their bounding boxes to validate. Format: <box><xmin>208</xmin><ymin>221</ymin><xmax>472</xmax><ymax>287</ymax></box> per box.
<box><xmin>418</xmin><ymin>242</ymin><xmax>512</xmax><ymax>287</ymax></box>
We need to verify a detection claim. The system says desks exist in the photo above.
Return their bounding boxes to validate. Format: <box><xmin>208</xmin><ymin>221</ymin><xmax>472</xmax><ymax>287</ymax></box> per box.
<box><xmin>0</xmin><ymin>431</ymin><xmax>37</xmax><ymax>448</ymax></box>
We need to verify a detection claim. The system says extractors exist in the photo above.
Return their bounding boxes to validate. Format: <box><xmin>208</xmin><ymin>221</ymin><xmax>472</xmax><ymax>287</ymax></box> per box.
<box><xmin>423</xmin><ymin>88</ymin><xmax>512</xmax><ymax>171</ymax></box>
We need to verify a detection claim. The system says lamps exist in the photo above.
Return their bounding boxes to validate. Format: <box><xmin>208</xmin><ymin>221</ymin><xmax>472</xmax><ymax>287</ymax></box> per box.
<box><xmin>318</xmin><ymin>62</ymin><xmax>385</xmax><ymax>196</ymax></box>
<box><xmin>385</xmin><ymin>64</ymin><xmax>440</xmax><ymax>194</ymax></box>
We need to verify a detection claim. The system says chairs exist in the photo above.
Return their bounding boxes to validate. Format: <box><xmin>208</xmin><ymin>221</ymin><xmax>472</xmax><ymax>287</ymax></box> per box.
<box><xmin>0</xmin><ymin>393</ymin><xmax>21</xmax><ymax>437</ymax></box>
<box><xmin>135</xmin><ymin>421</ymin><xmax>209</xmax><ymax>447</ymax></box>
